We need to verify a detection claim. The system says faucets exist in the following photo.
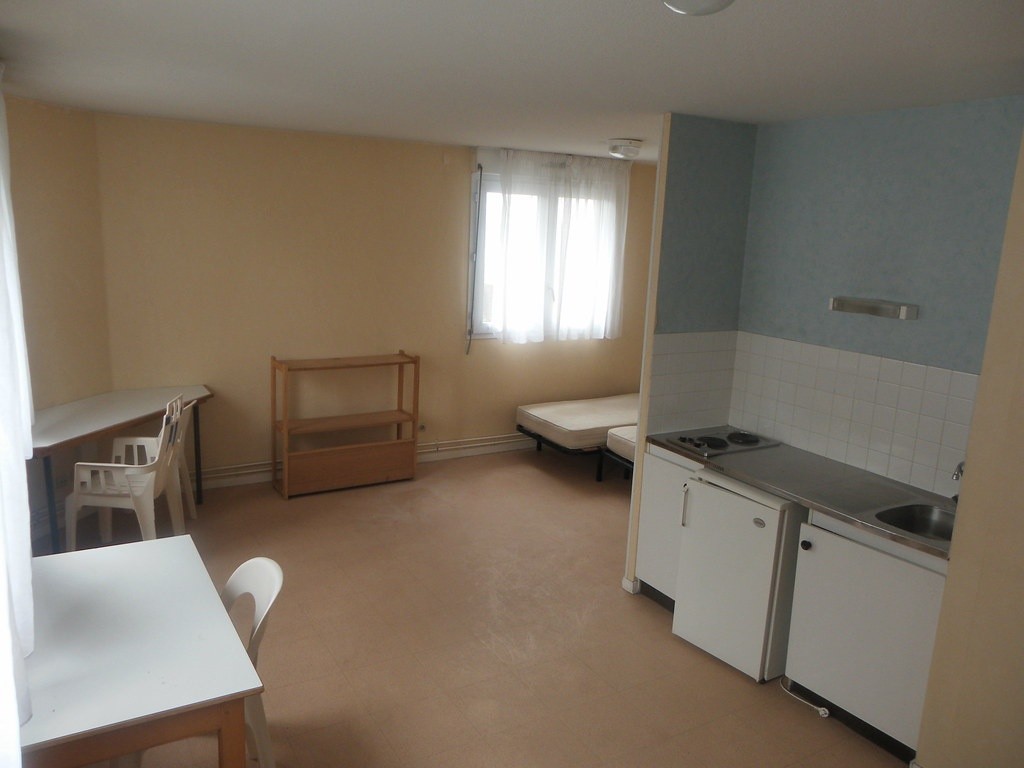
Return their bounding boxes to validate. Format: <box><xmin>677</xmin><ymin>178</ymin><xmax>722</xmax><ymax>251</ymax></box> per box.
<box><xmin>951</xmin><ymin>461</ymin><xmax>965</xmax><ymax>480</ymax></box>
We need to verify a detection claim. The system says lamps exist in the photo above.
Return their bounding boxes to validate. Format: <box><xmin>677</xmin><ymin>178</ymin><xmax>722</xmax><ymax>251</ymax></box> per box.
<box><xmin>607</xmin><ymin>140</ymin><xmax>643</xmax><ymax>160</ymax></box>
<box><xmin>662</xmin><ymin>0</ymin><xmax>735</xmax><ymax>16</ymax></box>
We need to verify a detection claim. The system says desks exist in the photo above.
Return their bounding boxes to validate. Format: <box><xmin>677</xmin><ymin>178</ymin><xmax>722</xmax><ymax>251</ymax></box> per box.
<box><xmin>20</xmin><ymin>535</ymin><xmax>266</xmax><ymax>768</ymax></box>
<box><xmin>29</xmin><ymin>384</ymin><xmax>215</xmax><ymax>555</ymax></box>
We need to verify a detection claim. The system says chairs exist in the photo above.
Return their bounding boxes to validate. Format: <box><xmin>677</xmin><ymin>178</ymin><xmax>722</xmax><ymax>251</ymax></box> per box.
<box><xmin>64</xmin><ymin>393</ymin><xmax>198</xmax><ymax>541</ymax></box>
<box><xmin>111</xmin><ymin>557</ymin><xmax>284</xmax><ymax>768</ymax></box>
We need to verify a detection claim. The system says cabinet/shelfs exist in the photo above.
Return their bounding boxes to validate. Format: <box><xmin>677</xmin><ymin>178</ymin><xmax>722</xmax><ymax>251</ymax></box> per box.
<box><xmin>786</xmin><ymin>509</ymin><xmax>949</xmax><ymax>766</ymax></box>
<box><xmin>269</xmin><ymin>347</ymin><xmax>419</xmax><ymax>501</ymax></box>
<box><xmin>635</xmin><ymin>443</ymin><xmax>808</xmax><ymax>686</ymax></box>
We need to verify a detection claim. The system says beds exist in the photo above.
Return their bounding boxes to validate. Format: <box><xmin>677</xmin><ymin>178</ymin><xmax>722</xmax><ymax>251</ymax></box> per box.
<box><xmin>512</xmin><ymin>391</ymin><xmax>640</xmax><ymax>484</ymax></box>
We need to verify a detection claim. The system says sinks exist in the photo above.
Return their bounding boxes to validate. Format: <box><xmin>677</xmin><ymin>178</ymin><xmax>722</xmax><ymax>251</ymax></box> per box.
<box><xmin>874</xmin><ymin>503</ymin><xmax>955</xmax><ymax>543</ymax></box>
